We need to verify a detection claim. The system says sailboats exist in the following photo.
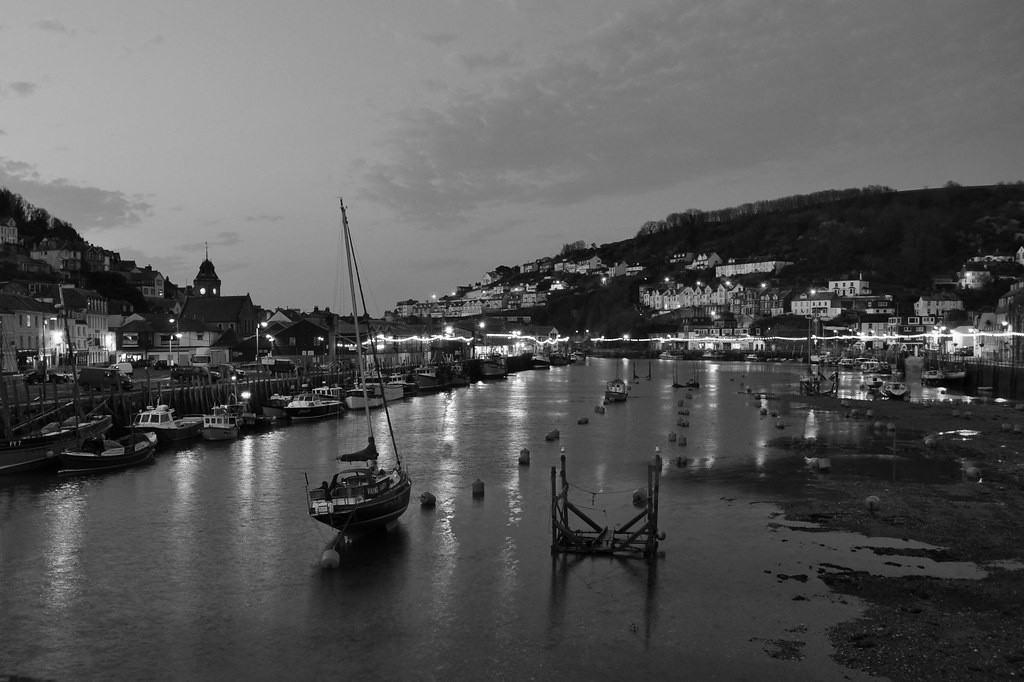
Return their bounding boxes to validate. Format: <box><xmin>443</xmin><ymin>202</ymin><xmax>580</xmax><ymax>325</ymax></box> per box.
<box><xmin>301</xmin><ymin>197</ymin><xmax>410</xmax><ymax>545</ymax></box>
<box><xmin>603</xmin><ymin>359</ymin><xmax>628</xmax><ymax>402</ymax></box>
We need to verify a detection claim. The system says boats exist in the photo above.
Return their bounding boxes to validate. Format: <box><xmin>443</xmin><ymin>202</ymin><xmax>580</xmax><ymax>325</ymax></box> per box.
<box><xmin>657</xmin><ymin>327</ymin><xmax>973</xmax><ymax>408</ymax></box>
<box><xmin>0</xmin><ymin>299</ymin><xmax>590</xmax><ymax>480</ymax></box>
